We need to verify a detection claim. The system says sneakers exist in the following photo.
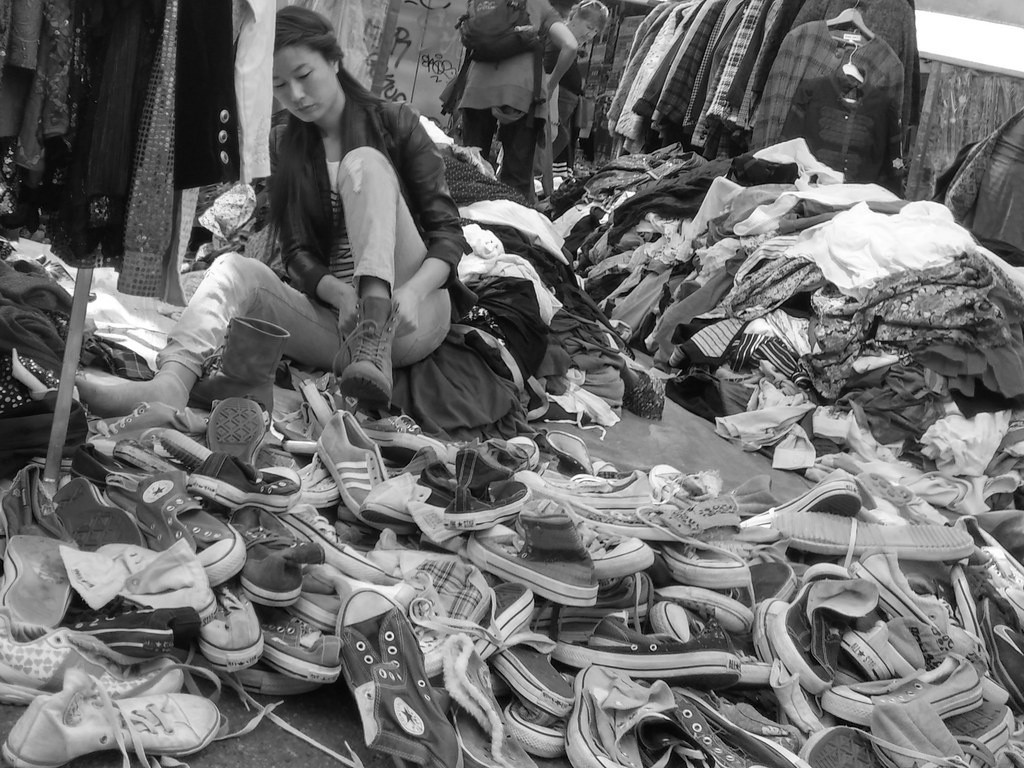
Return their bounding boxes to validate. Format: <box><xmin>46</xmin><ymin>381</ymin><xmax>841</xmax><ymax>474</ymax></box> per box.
<box><xmin>0</xmin><ymin>294</ymin><xmax>1024</xmax><ymax>768</ymax></box>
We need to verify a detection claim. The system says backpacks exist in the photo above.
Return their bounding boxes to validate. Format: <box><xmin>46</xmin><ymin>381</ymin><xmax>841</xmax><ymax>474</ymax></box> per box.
<box><xmin>455</xmin><ymin>0</ymin><xmax>544</xmax><ymax>63</ymax></box>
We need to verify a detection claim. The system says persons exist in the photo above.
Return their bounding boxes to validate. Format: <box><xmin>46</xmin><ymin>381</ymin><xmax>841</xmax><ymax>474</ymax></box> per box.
<box><xmin>458</xmin><ymin>0</ymin><xmax>578</xmax><ymax>201</ymax></box>
<box><xmin>536</xmin><ymin>0</ymin><xmax>610</xmax><ymax>164</ymax></box>
<box><xmin>79</xmin><ymin>6</ymin><xmax>478</xmax><ymax>422</ymax></box>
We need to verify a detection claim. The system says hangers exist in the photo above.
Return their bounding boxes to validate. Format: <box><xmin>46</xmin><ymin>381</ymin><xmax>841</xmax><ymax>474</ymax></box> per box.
<box><xmin>827</xmin><ymin>0</ymin><xmax>874</xmax><ymax>41</ymax></box>
<box><xmin>840</xmin><ymin>41</ymin><xmax>863</xmax><ymax>83</ymax></box>
